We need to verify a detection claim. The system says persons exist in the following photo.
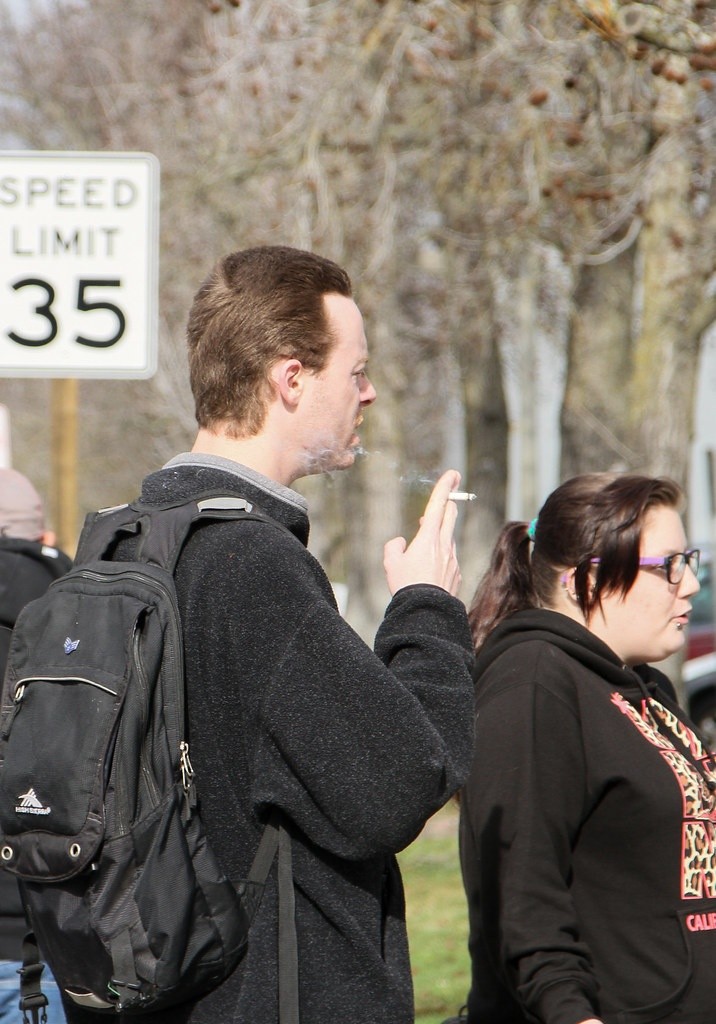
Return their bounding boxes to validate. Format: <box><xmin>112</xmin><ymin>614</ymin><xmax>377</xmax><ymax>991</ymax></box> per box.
<box><xmin>455</xmin><ymin>472</ymin><xmax>716</xmax><ymax>1024</ymax></box>
<box><xmin>0</xmin><ymin>464</ymin><xmax>67</xmax><ymax>1024</ymax></box>
<box><xmin>100</xmin><ymin>246</ymin><xmax>475</xmax><ymax>1024</ymax></box>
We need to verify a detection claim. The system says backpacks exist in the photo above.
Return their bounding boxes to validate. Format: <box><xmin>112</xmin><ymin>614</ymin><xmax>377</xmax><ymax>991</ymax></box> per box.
<box><xmin>1</xmin><ymin>536</ymin><xmax>75</xmax><ymax>709</ymax></box>
<box><xmin>0</xmin><ymin>488</ymin><xmax>306</xmax><ymax>1017</ymax></box>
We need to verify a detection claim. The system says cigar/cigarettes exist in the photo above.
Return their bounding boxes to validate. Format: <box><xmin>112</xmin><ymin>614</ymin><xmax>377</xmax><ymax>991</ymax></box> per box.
<box><xmin>448</xmin><ymin>492</ymin><xmax>477</xmax><ymax>500</ymax></box>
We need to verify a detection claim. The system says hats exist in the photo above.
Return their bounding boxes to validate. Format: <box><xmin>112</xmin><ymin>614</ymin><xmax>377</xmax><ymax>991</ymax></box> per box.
<box><xmin>0</xmin><ymin>468</ymin><xmax>45</xmax><ymax>542</ymax></box>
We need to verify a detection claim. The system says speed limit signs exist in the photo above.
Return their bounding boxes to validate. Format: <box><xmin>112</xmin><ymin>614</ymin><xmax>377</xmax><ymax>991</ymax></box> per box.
<box><xmin>0</xmin><ymin>150</ymin><xmax>166</xmax><ymax>383</ymax></box>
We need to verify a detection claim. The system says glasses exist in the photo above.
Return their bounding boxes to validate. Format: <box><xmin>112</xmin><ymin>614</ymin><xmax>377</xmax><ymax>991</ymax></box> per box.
<box><xmin>558</xmin><ymin>549</ymin><xmax>701</xmax><ymax>587</ymax></box>
